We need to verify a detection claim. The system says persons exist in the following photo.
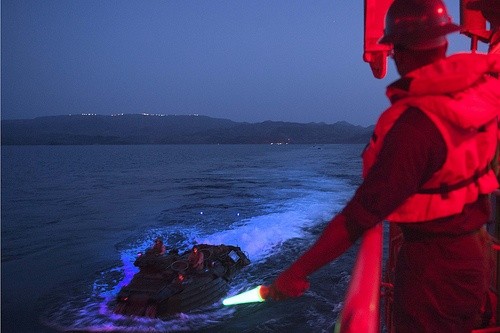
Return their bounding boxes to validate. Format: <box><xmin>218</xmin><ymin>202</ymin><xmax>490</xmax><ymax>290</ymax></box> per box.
<box><xmin>271</xmin><ymin>0</ymin><xmax>500</xmax><ymax>333</ymax></box>
<box><xmin>151</xmin><ymin>238</ymin><xmax>166</xmax><ymax>256</ymax></box>
<box><xmin>462</xmin><ymin>0</ymin><xmax>500</xmax><ymax>55</ymax></box>
<box><xmin>187</xmin><ymin>245</ymin><xmax>206</xmax><ymax>272</ymax></box>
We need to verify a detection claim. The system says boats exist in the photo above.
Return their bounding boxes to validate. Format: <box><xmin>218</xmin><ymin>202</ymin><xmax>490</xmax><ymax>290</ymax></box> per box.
<box><xmin>114</xmin><ymin>243</ymin><xmax>251</xmax><ymax>320</ymax></box>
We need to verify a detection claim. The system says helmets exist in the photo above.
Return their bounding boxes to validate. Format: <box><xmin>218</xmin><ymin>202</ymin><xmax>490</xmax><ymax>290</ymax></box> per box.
<box><xmin>378</xmin><ymin>0</ymin><xmax>459</xmax><ymax>47</ymax></box>
